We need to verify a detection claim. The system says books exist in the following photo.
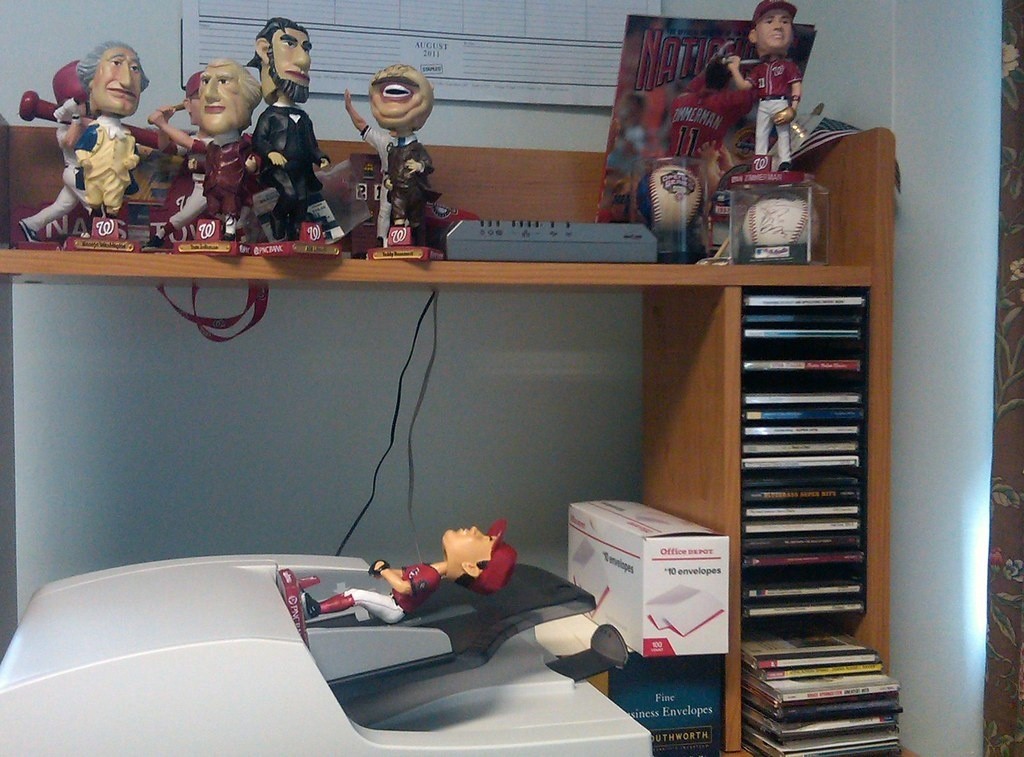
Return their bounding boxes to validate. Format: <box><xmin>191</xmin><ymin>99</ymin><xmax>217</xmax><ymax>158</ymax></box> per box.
<box><xmin>741</xmin><ymin>294</ymin><xmax>904</xmax><ymax>755</ymax></box>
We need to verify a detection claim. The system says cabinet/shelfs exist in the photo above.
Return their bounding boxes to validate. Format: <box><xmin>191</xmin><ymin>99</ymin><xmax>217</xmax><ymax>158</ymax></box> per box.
<box><xmin>0</xmin><ymin>110</ymin><xmax>919</xmax><ymax>757</ymax></box>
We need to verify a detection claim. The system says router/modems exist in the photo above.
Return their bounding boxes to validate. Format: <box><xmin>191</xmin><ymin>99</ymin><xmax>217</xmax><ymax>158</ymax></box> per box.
<box><xmin>437</xmin><ymin>219</ymin><xmax>657</xmax><ymax>262</ymax></box>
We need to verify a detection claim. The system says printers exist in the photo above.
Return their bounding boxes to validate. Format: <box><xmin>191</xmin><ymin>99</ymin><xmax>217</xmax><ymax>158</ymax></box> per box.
<box><xmin>2</xmin><ymin>553</ymin><xmax>653</xmax><ymax>757</ymax></box>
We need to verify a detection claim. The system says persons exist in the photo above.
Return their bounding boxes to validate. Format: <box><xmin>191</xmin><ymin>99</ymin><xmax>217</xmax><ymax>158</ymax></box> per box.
<box><xmin>666</xmin><ymin>34</ymin><xmax>756</xmax><ymax>169</ymax></box>
